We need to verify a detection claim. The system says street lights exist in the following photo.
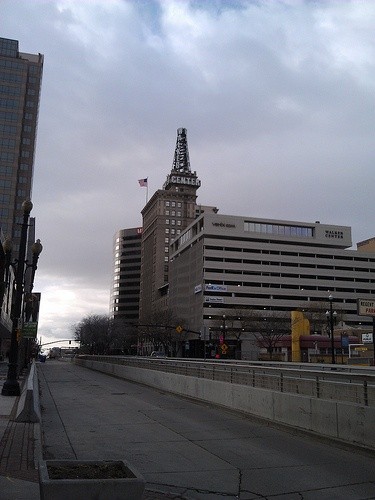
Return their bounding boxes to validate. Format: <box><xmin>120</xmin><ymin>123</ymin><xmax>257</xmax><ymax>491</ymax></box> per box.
<box><xmin>0</xmin><ymin>198</ymin><xmax>43</xmax><ymax>397</ymax></box>
<box><xmin>326</xmin><ymin>294</ymin><xmax>338</xmax><ymax>371</ymax></box>
<box><xmin>220</xmin><ymin>314</ymin><xmax>229</xmax><ymax>348</ymax></box>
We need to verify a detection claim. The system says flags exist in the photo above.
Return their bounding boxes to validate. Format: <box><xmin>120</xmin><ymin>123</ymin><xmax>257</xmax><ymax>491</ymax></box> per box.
<box><xmin>138</xmin><ymin>178</ymin><xmax>147</xmax><ymax>186</ymax></box>
